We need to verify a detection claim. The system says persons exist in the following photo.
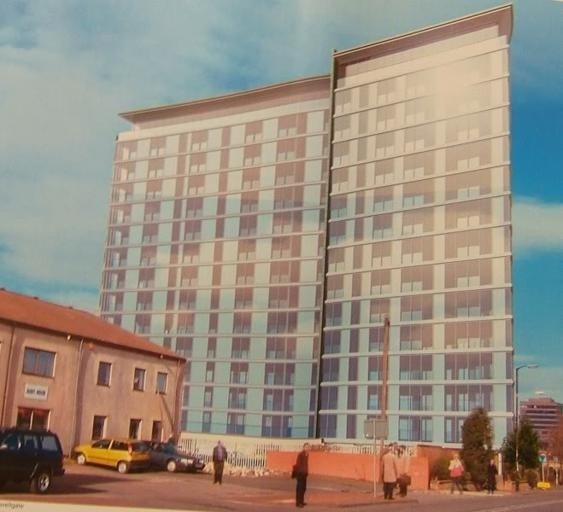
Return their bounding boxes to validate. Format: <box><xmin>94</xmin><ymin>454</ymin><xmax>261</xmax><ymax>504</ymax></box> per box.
<box><xmin>211</xmin><ymin>439</ymin><xmax>228</xmax><ymax>486</ymax></box>
<box><xmin>486</xmin><ymin>458</ymin><xmax>497</xmax><ymax>494</ymax></box>
<box><xmin>378</xmin><ymin>441</ymin><xmax>409</xmax><ymax>501</ymax></box>
<box><xmin>447</xmin><ymin>452</ymin><xmax>465</xmax><ymax>496</ymax></box>
<box><xmin>293</xmin><ymin>442</ymin><xmax>310</xmax><ymax>505</ymax></box>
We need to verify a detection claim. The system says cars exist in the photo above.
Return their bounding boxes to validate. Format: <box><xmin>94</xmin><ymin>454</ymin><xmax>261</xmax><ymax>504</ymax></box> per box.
<box><xmin>68</xmin><ymin>434</ymin><xmax>152</xmax><ymax>475</ymax></box>
<box><xmin>139</xmin><ymin>436</ymin><xmax>205</xmax><ymax>477</ymax></box>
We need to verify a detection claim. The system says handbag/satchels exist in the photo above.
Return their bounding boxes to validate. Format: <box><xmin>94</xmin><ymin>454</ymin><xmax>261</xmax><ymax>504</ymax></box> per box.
<box><xmin>395</xmin><ymin>473</ymin><xmax>411</xmax><ymax>486</ymax></box>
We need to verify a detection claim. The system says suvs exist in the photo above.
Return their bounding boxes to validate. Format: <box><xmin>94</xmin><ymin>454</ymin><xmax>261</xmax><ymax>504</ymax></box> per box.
<box><xmin>0</xmin><ymin>423</ymin><xmax>72</xmax><ymax>495</ymax></box>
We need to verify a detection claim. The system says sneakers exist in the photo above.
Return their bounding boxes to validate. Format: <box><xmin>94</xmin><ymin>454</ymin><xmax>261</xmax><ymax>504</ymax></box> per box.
<box><xmin>295</xmin><ymin>501</ymin><xmax>307</xmax><ymax>508</ymax></box>
<box><xmin>382</xmin><ymin>492</ymin><xmax>407</xmax><ymax>499</ymax></box>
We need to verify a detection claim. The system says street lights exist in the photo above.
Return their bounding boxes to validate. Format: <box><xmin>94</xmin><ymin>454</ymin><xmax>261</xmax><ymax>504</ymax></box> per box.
<box><xmin>363</xmin><ymin>432</ymin><xmax>377</xmax><ymax>499</ymax></box>
<box><xmin>514</xmin><ymin>363</ymin><xmax>540</xmax><ymax>472</ymax></box>
